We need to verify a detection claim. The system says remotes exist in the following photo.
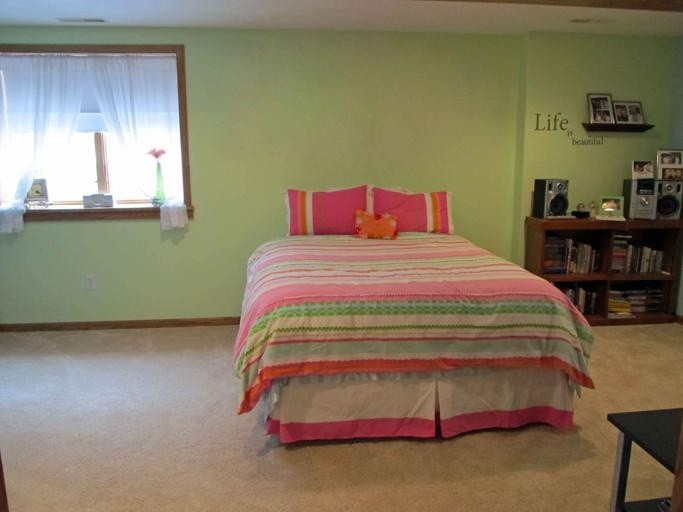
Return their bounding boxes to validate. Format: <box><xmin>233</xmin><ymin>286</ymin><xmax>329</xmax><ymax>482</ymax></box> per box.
<box><xmin>545</xmin><ymin>216</ymin><xmax>576</xmax><ymax>219</ymax></box>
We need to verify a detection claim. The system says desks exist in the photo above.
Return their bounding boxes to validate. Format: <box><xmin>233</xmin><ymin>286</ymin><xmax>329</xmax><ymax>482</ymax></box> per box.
<box><xmin>606</xmin><ymin>407</ymin><xmax>683</xmax><ymax>511</ymax></box>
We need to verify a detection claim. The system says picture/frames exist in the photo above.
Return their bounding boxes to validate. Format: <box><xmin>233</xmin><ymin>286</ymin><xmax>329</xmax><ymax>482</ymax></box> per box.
<box><xmin>587</xmin><ymin>91</ymin><xmax>646</xmax><ymax>125</ymax></box>
<box><xmin>18</xmin><ymin>178</ymin><xmax>49</xmax><ymax>204</ymax></box>
<box><xmin>630</xmin><ymin>149</ymin><xmax>682</xmax><ymax>181</ymax></box>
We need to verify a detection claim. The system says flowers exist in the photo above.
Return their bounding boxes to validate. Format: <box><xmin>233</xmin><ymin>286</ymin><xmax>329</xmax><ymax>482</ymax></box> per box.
<box><xmin>147</xmin><ymin>144</ymin><xmax>167</xmax><ymax>162</ymax></box>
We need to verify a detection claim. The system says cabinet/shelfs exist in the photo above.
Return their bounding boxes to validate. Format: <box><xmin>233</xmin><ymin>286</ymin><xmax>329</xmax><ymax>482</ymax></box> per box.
<box><xmin>524</xmin><ymin>216</ymin><xmax>683</xmax><ymax>326</ymax></box>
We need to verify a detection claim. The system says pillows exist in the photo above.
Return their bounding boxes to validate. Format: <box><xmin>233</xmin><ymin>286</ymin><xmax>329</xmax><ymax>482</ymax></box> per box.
<box><xmin>284</xmin><ymin>186</ymin><xmax>454</xmax><ymax>240</ymax></box>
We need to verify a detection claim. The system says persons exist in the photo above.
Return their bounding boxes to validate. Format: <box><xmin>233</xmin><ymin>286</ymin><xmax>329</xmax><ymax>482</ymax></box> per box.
<box><xmin>660</xmin><ymin>152</ymin><xmax>682</xmax><ymax>181</ymax></box>
<box><xmin>634</xmin><ymin>161</ymin><xmax>653</xmax><ymax>179</ymax></box>
<box><xmin>615</xmin><ymin>105</ymin><xmax>642</xmax><ymax>122</ymax></box>
<box><xmin>591</xmin><ymin>97</ymin><xmax>611</xmax><ymax>123</ymax></box>
<box><xmin>601</xmin><ymin>199</ymin><xmax>619</xmax><ymax>212</ymax></box>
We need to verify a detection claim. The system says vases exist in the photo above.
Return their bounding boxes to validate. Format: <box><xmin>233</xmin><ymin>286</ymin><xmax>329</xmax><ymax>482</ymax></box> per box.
<box><xmin>153</xmin><ymin>162</ymin><xmax>169</xmax><ymax>205</ymax></box>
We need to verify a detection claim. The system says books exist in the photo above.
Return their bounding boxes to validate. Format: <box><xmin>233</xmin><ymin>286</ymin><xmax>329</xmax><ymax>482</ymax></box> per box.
<box><xmin>544</xmin><ymin>235</ymin><xmax>664</xmax><ymax>321</ymax></box>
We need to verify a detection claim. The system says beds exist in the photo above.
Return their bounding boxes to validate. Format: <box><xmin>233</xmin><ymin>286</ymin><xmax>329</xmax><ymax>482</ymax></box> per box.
<box><xmin>239</xmin><ymin>231</ymin><xmax>578</xmax><ymax>442</ymax></box>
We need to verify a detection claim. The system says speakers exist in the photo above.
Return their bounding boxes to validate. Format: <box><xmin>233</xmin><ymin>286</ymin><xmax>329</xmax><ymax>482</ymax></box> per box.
<box><xmin>534</xmin><ymin>179</ymin><xmax>568</xmax><ymax>220</ymax></box>
<box><xmin>655</xmin><ymin>180</ymin><xmax>683</xmax><ymax>220</ymax></box>
